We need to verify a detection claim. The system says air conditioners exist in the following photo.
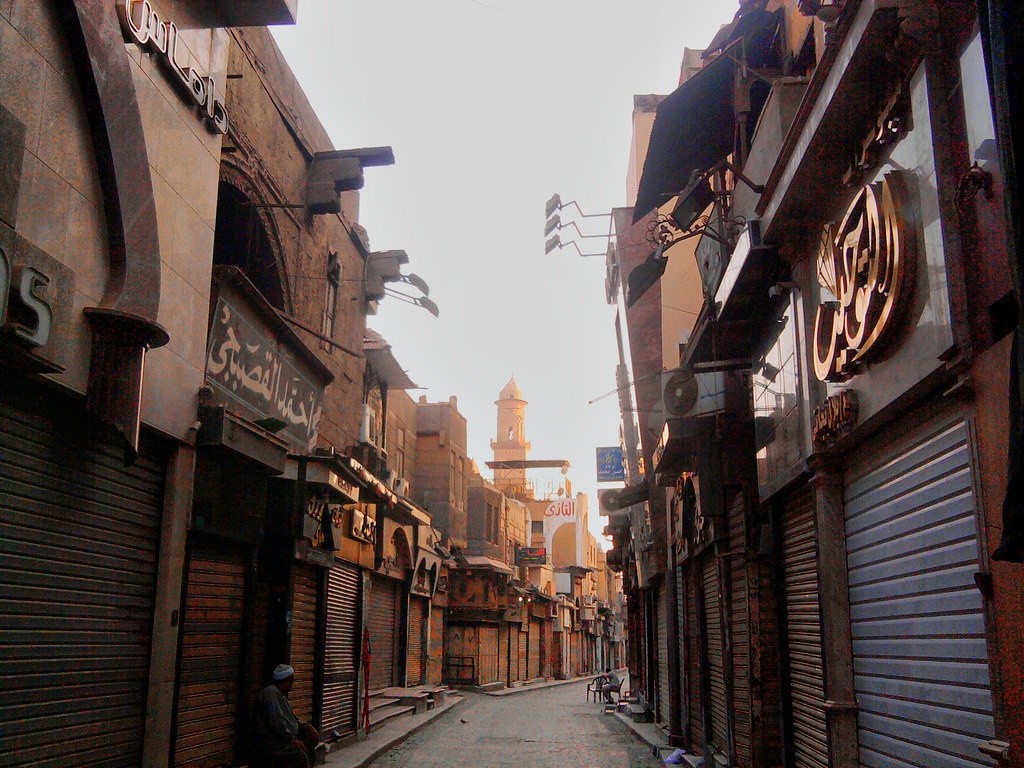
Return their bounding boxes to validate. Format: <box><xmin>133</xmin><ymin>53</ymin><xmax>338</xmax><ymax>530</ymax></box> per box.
<box><xmin>598</xmin><ymin>489</ymin><xmax>628</xmax><ymax>516</ymax></box>
<box><xmin>660</xmin><ymin>371</ymin><xmax>743</xmax><ymax>422</ymax></box>
<box><xmin>397</xmin><ymin>478</ymin><xmax>409</xmax><ymax>497</ymax></box>
<box><xmin>385</xmin><ymin>469</ymin><xmax>398</xmax><ymax>491</ymax></box>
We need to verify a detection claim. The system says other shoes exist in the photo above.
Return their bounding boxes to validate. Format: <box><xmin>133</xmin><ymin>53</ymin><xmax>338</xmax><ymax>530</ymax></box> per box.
<box><xmin>606</xmin><ymin>700</ymin><xmax>614</xmax><ymax>704</ymax></box>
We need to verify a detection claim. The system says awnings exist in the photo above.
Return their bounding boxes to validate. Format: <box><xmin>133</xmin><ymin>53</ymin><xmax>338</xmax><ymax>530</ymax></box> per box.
<box><xmin>632</xmin><ymin>28</ymin><xmax>758</xmax><ymax>224</ymax></box>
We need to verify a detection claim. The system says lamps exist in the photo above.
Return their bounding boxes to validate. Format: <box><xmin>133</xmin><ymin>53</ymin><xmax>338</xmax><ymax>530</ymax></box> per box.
<box><xmin>624</xmin><ymin>213</ymin><xmax>747</xmax><ymax>309</ymax></box>
<box><xmin>670</xmin><ymin>158</ymin><xmax>764</xmax><ymax>234</ymax></box>
<box><xmin>433</xmin><ymin>542</ymin><xmax>452</xmax><ymax>560</ymax></box>
<box><xmin>544</xmin><ymin>193</ymin><xmax>612</xmax><ymax>257</ymax></box>
<box><xmin>384</xmin><ymin>286</ymin><xmax>439</xmax><ymax>318</ymax></box>
<box><xmin>399</xmin><ymin>273</ymin><xmax>430</xmax><ymax>297</ymax></box>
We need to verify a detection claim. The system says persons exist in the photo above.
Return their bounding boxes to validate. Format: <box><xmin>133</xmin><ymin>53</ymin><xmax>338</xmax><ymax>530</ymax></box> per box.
<box><xmin>257</xmin><ymin>664</ymin><xmax>321</xmax><ymax>768</ymax></box>
<box><xmin>601</xmin><ymin>668</ymin><xmax>619</xmax><ymax>704</ymax></box>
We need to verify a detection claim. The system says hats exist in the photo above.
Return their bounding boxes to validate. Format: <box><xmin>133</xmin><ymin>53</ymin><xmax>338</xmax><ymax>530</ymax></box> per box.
<box><xmin>273</xmin><ymin>664</ymin><xmax>294</xmax><ymax>679</ymax></box>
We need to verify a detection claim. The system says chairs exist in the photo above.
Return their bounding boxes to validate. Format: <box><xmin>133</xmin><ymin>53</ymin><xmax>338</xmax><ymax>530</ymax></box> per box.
<box><xmin>587</xmin><ymin>676</ymin><xmax>608</xmax><ymax>704</ymax></box>
<box><xmin>605</xmin><ymin>679</ymin><xmax>625</xmax><ymax>704</ymax></box>
